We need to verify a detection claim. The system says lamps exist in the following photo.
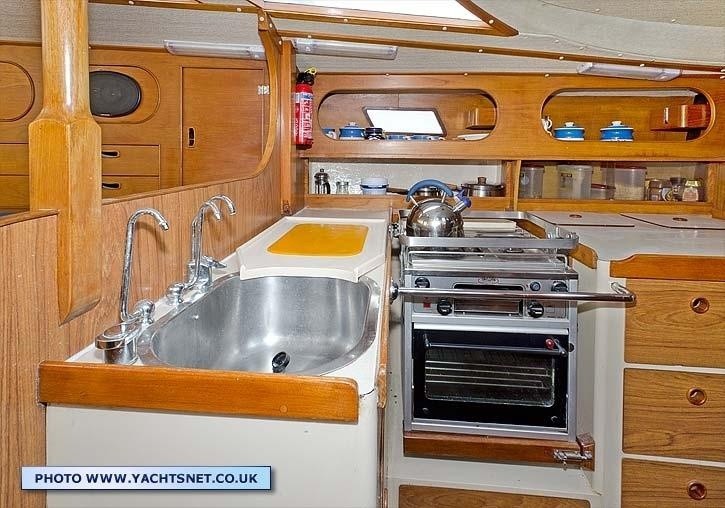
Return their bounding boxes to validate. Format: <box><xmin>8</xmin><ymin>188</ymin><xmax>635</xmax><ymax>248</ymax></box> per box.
<box><xmin>577</xmin><ymin>61</ymin><xmax>682</xmax><ymax>82</ymax></box>
<box><xmin>293</xmin><ymin>37</ymin><xmax>400</xmax><ymax>60</ymax></box>
<box><xmin>163</xmin><ymin>39</ymin><xmax>265</xmax><ymax>60</ymax></box>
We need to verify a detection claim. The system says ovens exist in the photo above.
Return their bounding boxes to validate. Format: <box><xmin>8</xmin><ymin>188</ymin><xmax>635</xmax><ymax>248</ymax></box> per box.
<box><xmin>399</xmin><ymin>322</ymin><xmax>579</xmax><ymax>443</ymax></box>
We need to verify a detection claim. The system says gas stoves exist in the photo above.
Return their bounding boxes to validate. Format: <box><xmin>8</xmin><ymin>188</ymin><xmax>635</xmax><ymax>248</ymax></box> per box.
<box><xmin>398</xmin><ymin>238</ymin><xmax>579</xmax><ymax>321</ymax></box>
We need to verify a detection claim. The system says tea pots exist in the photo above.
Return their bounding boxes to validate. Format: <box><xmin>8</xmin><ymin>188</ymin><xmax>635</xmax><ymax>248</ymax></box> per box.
<box><xmin>404</xmin><ymin>179</ymin><xmax>472</xmax><ymax>238</ymax></box>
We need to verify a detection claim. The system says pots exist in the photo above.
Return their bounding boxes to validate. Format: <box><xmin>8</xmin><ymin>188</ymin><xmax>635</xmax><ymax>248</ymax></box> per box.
<box><xmin>414</xmin><ymin>184</ymin><xmax>443</xmax><ymax>197</ymax></box>
<box><xmin>460</xmin><ymin>177</ymin><xmax>506</xmax><ymax>197</ymax></box>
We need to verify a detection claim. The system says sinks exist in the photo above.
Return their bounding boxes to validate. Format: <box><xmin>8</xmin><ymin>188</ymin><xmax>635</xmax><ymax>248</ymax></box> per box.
<box><xmin>133</xmin><ymin>268</ymin><xmax>380</xmax><ymax>376</ymax></box>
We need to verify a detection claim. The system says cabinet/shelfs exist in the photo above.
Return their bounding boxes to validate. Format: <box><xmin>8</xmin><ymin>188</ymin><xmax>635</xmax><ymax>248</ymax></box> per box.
<box><xmin>291</xmin><ymin>70</ymin><xmax>724</xmax><ymax>219</ymax></box>
<box><xmin>622</xmin><ymin>280</ymin><xmax>725</xmax><ymax>506</ymax></box>
<box><xmin>183</xmin><ymin>69</ymin><xmax>269</xmax><ymax>185</ymax></box>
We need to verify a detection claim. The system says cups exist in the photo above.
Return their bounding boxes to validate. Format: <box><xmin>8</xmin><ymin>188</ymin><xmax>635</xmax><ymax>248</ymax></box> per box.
<box><xmin>314</xmin><ymin>168</ymin><xmax>330</xmax><ymax>194</ymax></box>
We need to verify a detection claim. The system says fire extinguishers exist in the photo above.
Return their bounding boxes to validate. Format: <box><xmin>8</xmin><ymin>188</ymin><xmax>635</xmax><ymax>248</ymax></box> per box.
<box><xmin>294</xmin><ymin>71</ymin><xmax>314</xmax><ymax>150</ymax></box>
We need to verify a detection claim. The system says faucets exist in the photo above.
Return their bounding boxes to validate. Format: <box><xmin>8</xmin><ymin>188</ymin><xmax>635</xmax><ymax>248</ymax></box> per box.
<box><xmin>166</xmin><ymin>196</ymin><xmax>225</xmax><ymax>306</ymax></box>
<box><xmin>113</xmin><ymin>206</ymin><xmax>170</xmax><ymax>321</ymax></box>
<box><xmin>187</xmin><ymin>190</ymin><xmax>238</xmax><ymax>275</ymax></box>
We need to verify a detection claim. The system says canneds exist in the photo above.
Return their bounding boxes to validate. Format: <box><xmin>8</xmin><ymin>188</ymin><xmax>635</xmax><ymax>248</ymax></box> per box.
<box><xmin>646</xmin><ymin>179</ymin><xmax>673</xmax><ymax>201</ymax></box>
<box><xmin>670</xmin><ymin>176</ymin><xmax>686</xmax><ymax>201</ymax></box>
<box><xmin>681</xmin><ymin>178</ymin><xmax>706</xmax><ymax>202</ymax></box>
